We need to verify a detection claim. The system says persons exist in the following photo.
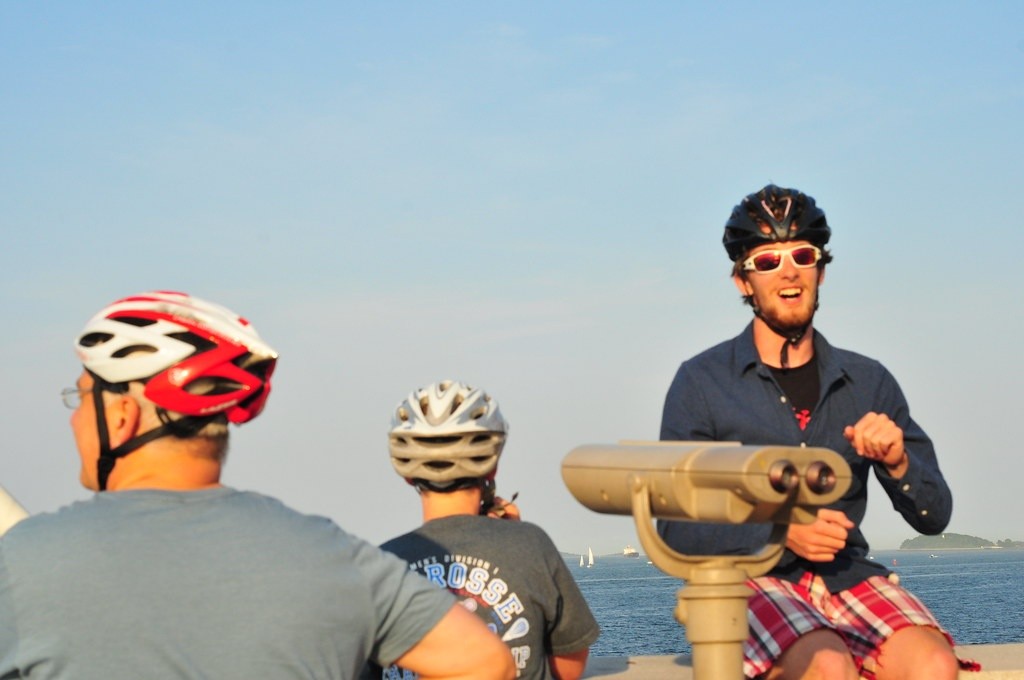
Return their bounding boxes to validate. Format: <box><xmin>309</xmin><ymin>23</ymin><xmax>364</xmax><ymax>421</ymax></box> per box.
<box><xmin>658</xmin><ymin>185</ymin><xmax>960</xmax><ymax>680</ymax></box>
<box><xmin>0</xmin><ymin>291</ymin><xmax>517</xmax><ymax>679</ymax></box>
<box><xmin>365</xmin><ymin>383</ymin><xmax>599</xmax><ymax>679</ymax></box>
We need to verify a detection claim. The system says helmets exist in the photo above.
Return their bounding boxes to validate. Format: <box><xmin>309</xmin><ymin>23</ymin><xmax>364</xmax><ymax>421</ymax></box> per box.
<box><xmin>722</xmin><ymin>183</ymin><xmax>831</xmax><ymax>262</ymax></box>
<box><xmin>73</xmin><ymin>291</ymin><xmax>279</xmax><ymax>426</ymax></box>
<box><xmin>387</xmin><ymin>380</ymin><xmax>509</xmax><ymax>493</ymax></box>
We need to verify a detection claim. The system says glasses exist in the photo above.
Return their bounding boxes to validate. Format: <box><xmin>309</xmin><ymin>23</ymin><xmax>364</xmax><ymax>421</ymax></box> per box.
<box><xmin>63</xmin><ymin>387</ymin><xmax>117</xmax><ymax>410</ymax></box>
<box><xmin>741</xmin><ymin>244</ymin><xmax>823</xmax><ymax>275</ymax></box>
